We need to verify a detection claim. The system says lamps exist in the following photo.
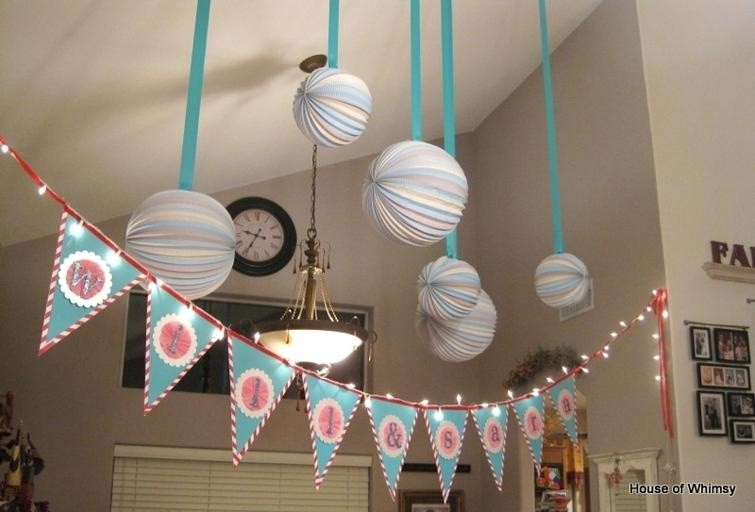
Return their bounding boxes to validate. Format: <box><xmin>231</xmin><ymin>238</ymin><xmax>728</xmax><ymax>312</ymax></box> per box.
<box><xmin>255</xmin><ymin>144</ymin><xmax>368</xmax><ymax>377</ymax></box>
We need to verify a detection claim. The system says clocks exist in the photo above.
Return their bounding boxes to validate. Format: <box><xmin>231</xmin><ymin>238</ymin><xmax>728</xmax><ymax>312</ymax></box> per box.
<box><xmin>224</xmin><ymin>197</ymin><xmax>297</xmax><ymax>278</ymax></box>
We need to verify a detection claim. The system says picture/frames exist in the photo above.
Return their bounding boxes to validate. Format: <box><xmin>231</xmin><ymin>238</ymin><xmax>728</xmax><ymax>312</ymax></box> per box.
<box><xmin>690</xmin><ymin>326</ymin><xmax>755</xmax><ymax>442</ymax></box>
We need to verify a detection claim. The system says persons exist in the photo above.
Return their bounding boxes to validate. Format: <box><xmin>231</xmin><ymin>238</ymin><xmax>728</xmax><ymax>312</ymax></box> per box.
<box><xmin>700</xmin><ymin>333</ymin><xmax>707</xmax><ymax>355</ymax></box>
<box><xmin>707</xmin><ymin>399</ymin><xmax>717</xmax><ymax>425</ymax></box>
<box><xmin>696</xmin><ymin>332</ymin><xmax>702</xmax><ymax>354</ymax></box>
<box><xmin>743</xmin><ymin>344</ymin><xmax>749</xmax><ymax>361</ymax></box>
<box><xmin>722</xmin><ymin>345</ymin><xmax>734</xmax><ymax>360</ymax></box>
<box><xmin>735</xmin><ymin>341</ymin><xmax>743</xmax><ymax>360</ymax></box>
<box><xmin>718</xmin><ymin>334</ymin><xmax>724</xmax><ymax>360</ymax></box>
